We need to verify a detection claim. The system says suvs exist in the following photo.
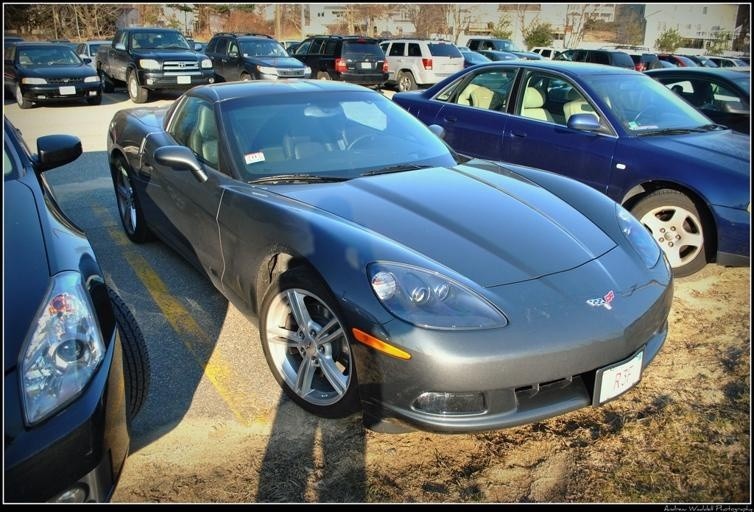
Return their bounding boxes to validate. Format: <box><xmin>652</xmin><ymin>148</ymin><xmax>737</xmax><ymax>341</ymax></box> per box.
<box><xmin>292</xmin><ymin>34</ymin><xmax>390</xmax><ymax>86</ymax></box>
<box><xmin>378</xmin><ymin>39</ymin><xmax>466</xmax><ymax>91</ymax></box>
<box><xmin>206</xmin><ymin>32</ymin><xmax>312</xmax><ymax>82</ymax></box>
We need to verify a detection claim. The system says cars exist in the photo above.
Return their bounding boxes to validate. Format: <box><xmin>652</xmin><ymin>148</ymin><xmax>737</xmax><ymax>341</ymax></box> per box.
<box><xmin>5</xmin><ymin>42</ymin><xmax>102</xmax><ymax>109</ymax></box>
<box><xmin>455</xmin><ymin>38</ymin><xmax>750</xmax><ymax>72</ymax></box>
<box><xmin>4</xmin><ymin>37</ymin><xmax>209</xmax><ymax>72</ymax></box>
<box><xmin>391</xmin><ymin>59</ymin><xmax>751</xmax><ymax>276</ymax></box>
<box><xmin>279</xmin><ymin>41</ymin><xmax>300</xmax><ymax>49</ymax></box>
<box><xmin>4</xmin><ymin>112</ymin><xmax>152</xmax><ymax>504</ymax></box>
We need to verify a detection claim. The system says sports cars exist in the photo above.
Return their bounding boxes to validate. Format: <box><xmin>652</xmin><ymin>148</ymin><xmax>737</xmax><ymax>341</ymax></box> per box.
<box><xmin>106</xmin><ymin>77</ymin><xmax>675</xmax><ymax>437</ymax></box>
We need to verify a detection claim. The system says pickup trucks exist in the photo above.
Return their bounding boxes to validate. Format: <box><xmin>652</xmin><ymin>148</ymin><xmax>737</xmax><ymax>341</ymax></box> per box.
<box><xmin>95</xmin><ymin>27</ymin><xmax>216</xmax><ymax>103</ymax></box>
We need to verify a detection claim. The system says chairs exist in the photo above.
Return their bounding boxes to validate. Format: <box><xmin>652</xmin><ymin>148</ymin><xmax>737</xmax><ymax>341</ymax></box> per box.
<box><xmin>564</xmin><ymin>88</ymin><xmax>613</xmax><ymax>135</ymax></box>
<box><xmin>189</xmin><ymin>103</ymin><xmax>239</xmax><ymax>165</ymax></box>
<box><xmin>470</xmin><ymin>85</ymin><xmax>499</xmax><ymax>110</ymax></box>
<box><xmin>671</xmin><ymin>85</ymin><xmax>685</xmax><ymax>93</ymax></box>
<box><xmin>521</xmin><ymin>86</ymin><xmax>556</xmax><ymax>121</ymax></box>
